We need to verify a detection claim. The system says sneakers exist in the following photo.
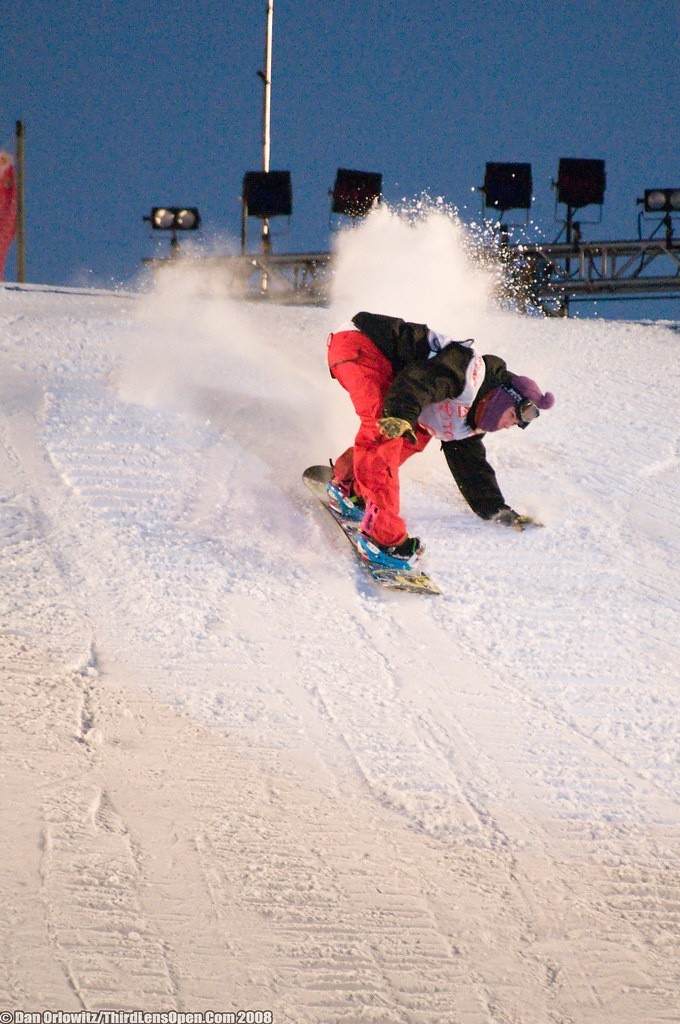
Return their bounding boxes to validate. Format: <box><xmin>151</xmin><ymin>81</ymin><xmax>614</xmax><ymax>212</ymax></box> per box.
<box><xmin>326</xmin><ymin>475</ymin><xmax>366</xmax><ymax>521</ymax></box>
<box><xmin>358</xmin><ymin>528</ymin><xmax>423</xmax><ymax>559</ymax></box>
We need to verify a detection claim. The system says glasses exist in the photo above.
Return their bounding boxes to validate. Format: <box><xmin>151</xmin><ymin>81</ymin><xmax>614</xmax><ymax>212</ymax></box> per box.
<box><xmin>502</xmin><ymin>383</ymin><xmax>540</xmax><ymax>423</ymax></box>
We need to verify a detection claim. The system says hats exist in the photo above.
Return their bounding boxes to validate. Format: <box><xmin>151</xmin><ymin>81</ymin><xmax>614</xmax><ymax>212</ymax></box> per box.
<box><xmin>474</xmin><ymin>375</ymin><xmax>555</xmax><ymax>432</ymax></box>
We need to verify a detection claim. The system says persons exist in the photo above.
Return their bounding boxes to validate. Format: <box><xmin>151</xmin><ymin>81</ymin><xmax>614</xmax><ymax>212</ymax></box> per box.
<box><xmin>327</xmin><ymin>310</ymin><xmax>555</xmax><ymax>558</ymax></box>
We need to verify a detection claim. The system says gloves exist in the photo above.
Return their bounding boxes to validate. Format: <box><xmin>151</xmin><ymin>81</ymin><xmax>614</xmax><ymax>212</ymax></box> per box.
<box><xmin>498</xmin><ymin>510</ymin><xmax>546</xmax><ymax>533</ymax></box>
<box><xmin>376</xmin><ymin>417</ymin><xmax>418</xmax><ymax>445</ymax></box>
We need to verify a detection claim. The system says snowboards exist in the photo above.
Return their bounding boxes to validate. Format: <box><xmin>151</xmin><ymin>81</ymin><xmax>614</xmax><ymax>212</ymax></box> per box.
<box><xmin>303</xmin><ymin>465</ymin><xmax>443</xmax><ymax>597</ymax></box>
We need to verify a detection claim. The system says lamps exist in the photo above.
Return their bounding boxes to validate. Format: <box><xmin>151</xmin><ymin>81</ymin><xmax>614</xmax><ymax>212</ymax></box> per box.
<box><xmin>544</xmin><ymin>156</ymin><xmax>607</xmax><ymax>227</ymax></box>
<box><xmin>471</xmin><ymin>159</ymin><xmax>535</xmax><ymax>228</ymax></box>
<box><xmin>142</xmin><ymin>205</ymin><xmax>203</xmax><ymax>259</ymax></box>
<box><xmin>242</xmin><ymin>170</ymin><xmax>294</xmax><ymax>254</ymax></box>
<box><xmin>635</xmin><ymin>186</ymin><xmax>680</xmax><ymax>251</ymax></box>
<box><xmin>325</xmin><ymin>168</ymin><xmax>384</xmax><ymax>233</ymax></box>
